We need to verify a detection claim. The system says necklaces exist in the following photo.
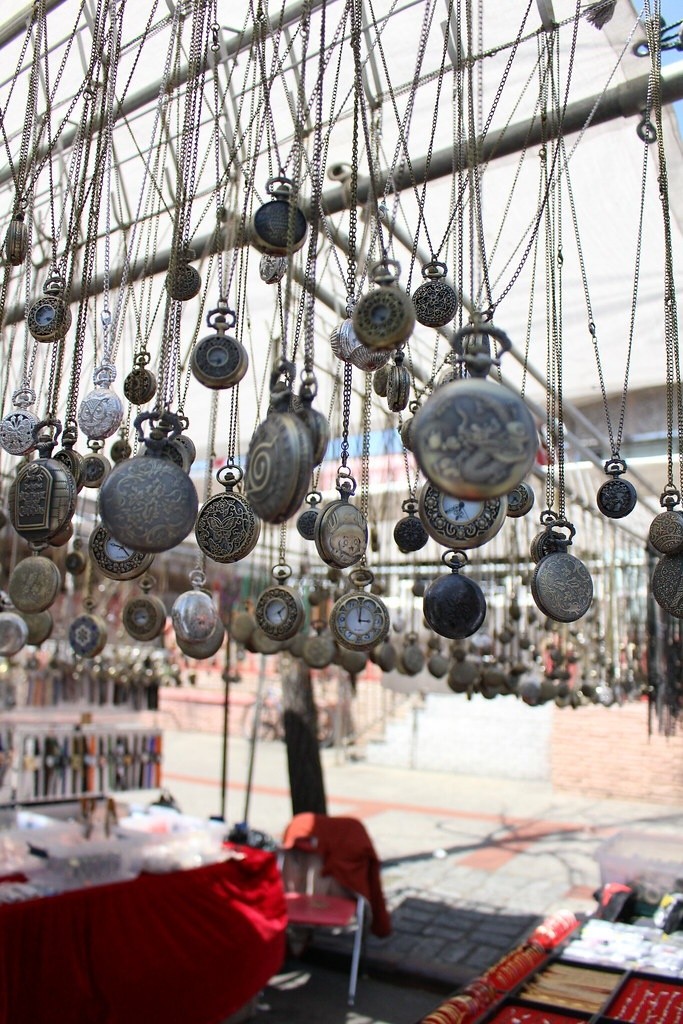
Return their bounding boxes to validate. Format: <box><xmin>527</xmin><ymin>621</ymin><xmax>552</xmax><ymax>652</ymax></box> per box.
<box><xmin>0</xmin><ymin>0</ymin><xmax>681</xmax><ymax>709</ymax></box>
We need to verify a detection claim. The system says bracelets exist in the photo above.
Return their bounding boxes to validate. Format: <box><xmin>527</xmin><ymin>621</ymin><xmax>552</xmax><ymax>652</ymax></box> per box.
<box><xmin>420</xmin><ymin>910</ymin><xmax>683</xmax><ymax>1024</ymax></box>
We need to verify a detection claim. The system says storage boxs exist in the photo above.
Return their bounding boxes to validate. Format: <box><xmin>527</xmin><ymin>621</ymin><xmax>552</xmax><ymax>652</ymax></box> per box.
<box><xmin>14</xmin><ymin>826</ymin><xmax>152</xmax><ymax>895</ymax></box>
<box><xmin>473</xmin><ymin>915</ymin><xmax>683</xmax><ymax>1024</ymax></box>
<box><xmin>114</xmin><ymin>814</ymin><xmax>229</xmax><ymax>875</ymax></box>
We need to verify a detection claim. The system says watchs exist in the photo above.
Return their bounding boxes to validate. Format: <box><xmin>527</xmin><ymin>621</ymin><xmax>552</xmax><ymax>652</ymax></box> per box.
<box><xmin>0</xmin><ymin>724</ymin><xmax>164</xmax><ymax>795</ymax></box>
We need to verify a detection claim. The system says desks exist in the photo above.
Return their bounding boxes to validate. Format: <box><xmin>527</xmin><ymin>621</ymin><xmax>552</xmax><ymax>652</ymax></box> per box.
<box><xmin>0</xmin><ymin>840</ymin><xmax>289</xmax><ymax>1024</ymax></box>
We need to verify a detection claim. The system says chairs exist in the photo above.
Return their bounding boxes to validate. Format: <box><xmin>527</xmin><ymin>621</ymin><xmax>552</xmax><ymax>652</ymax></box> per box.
<box><xmin>278</xmin><ymin>812</ymin><xmax>392</xmax><ymax>1006</ymax></box>
